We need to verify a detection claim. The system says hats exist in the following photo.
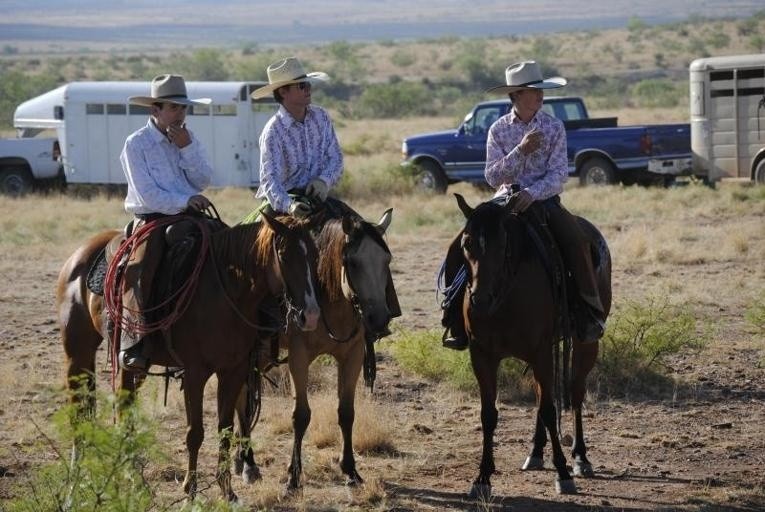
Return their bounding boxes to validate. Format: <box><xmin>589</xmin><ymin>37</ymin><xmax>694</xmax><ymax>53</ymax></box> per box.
<box><xmin>251</xmin><ymin>57</ymin><xmax>329</xmax><ymax>101</ymax></box>
<box><xmin>128</xmin><ymin>75</ymin><xmax>212</xmax><ymax>108</ymax></box>
<box><xmin>488</xmin><ymin>62</ymin><xmax>567</xmax><ymax>98</ymax></box>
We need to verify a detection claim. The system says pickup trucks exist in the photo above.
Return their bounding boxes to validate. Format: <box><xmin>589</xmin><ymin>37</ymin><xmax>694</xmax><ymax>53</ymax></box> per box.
<box><xmin>397</xmin><ymin>93</ymin><xmax>694</xmax><ymax>197</ymax></box>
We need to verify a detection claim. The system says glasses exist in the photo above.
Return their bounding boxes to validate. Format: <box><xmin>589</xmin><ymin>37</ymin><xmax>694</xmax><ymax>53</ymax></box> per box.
<box><xmin>288</xmin><ymin>82</ymin><xmax>306</xmax><ymax>89</ymax></box>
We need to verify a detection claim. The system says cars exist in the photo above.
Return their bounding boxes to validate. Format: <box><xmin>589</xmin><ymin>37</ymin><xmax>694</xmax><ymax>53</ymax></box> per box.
<box><xmin>0</xmin><ymin>135</ymin><xmax>64</xmax><ymax>197</ymax></box>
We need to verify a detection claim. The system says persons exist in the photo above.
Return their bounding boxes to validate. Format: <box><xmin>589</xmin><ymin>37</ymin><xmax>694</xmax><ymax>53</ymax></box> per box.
<box><xmin>440</xmin><ymin>61</ymin><xmax>606</xmax><ymax>350</ymax></box>
<box><xmin>250</xmin><ymin>54</ymin><xmax>346</xmax><ymax>331</ymax></box>
<box><xmin>118</xmin><ymin>75</ymin><xmax>214</xmax><ymax>371</ymax></box>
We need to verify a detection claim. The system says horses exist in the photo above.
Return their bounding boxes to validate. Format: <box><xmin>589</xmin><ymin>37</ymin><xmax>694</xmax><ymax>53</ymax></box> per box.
<box><xmin>452</xmin><ymin>191</ymin><xmax>615</xmax><ymax>500</ymax></box>
<box><xmin>232</xmin><ymin>199</ymin><xmax>396</xmax><ymax>503</ymax></box>
<box><xmin>51</xmin><ymin>205</ymin><xmax>330</xmax><ymax>512</ymax></box>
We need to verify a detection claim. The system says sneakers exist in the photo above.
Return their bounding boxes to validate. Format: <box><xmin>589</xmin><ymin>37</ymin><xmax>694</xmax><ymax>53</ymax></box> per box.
<box><xmin>444</xmin><ymin>328</ymin><xmax>465</xmax><ymax>348</ymax></box>
<box><xmin>580</xmin><ymin>321</ymin><xmax>601</xmax><ymax>344</ymax></box>
<box><xmin>124</xmin><ymin>344</ymin><xmax>145</xmax><ymax>369</ymax></box>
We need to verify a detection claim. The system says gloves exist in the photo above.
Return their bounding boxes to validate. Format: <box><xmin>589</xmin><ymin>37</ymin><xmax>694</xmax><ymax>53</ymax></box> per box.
<box><xmin>288</xmin><ymin>201</ymin><xmax>312</xmax><ymax>217</ymax></box>
<box><xmin>306</xmin><ymin>179</ymin><xmax>328</xmax><ymax>203</ymax></box>
